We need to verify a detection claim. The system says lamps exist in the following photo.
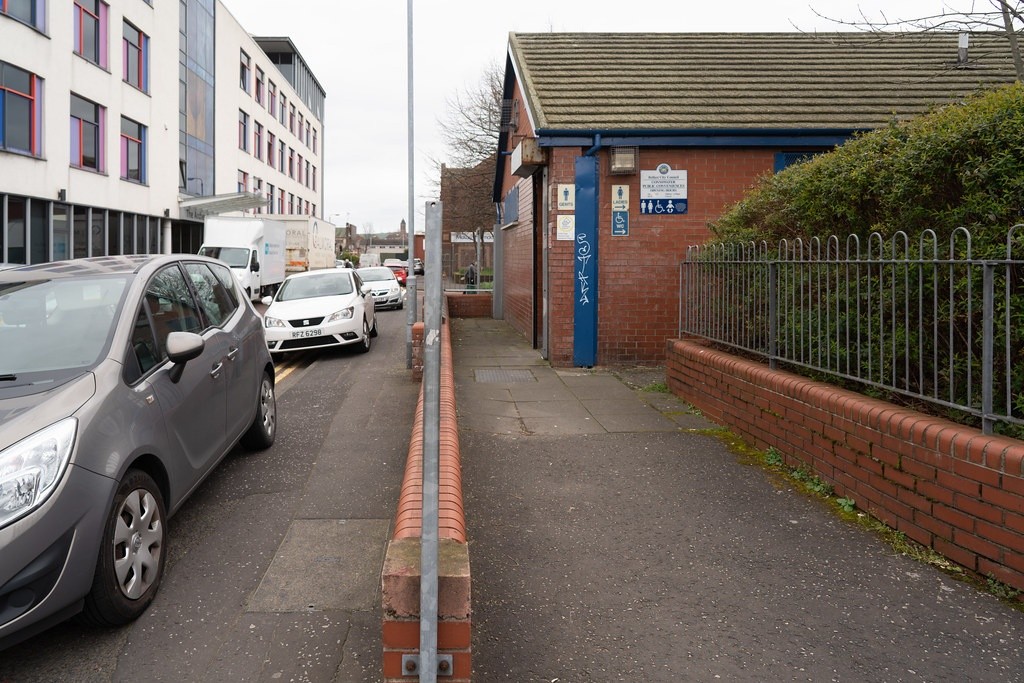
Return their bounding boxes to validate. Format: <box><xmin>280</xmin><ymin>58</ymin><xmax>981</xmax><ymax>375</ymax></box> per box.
<box><xmin>58</xmin><ymin>189</ymin><xmax>66</xmax><ymax>200</ymax></box>
<box><xmin>164</xmin><ymin>209</ymin><xmax>169</xmax><ymax>217</ymax></box>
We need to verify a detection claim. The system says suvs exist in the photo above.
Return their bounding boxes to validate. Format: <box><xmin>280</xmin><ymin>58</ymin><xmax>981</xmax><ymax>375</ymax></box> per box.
<box><xmin>0</xmin><ymin>253</ymin><xmax>278</xmax><ymax>651</ymax></box>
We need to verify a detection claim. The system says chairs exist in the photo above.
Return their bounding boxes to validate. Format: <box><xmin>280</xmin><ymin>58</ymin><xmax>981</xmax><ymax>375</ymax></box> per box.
<box><xmin>0</xmin><ymin>300</ymin><xmax>64</xmax><ymax>371</ymax></box>
<box><xmin>130</xmin><ymin>293</ymin><xmax>169</xmax><ymax>359</ymax></box>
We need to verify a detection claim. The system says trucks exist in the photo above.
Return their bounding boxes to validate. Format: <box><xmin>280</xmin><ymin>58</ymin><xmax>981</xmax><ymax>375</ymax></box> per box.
<box><xmin>196</xmin><ymin>219</ymin><xmax>287</xmax><ymax>303</ymax></box>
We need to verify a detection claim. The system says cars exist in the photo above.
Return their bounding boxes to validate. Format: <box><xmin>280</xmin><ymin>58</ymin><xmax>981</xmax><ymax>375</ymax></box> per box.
<box><xmin>260</xmin><ymin>269</ymin><xmax>378</xmax><ymax>361</ymax></box>
<box><xmin>354</xmin><ymin>266</ymin><xmax>403</xmax><ymax>310</ymax></box>
<box><xmin>383</xmin><ymin>258</ymin><xmax>424</xmax><ymax>287</ymax></box>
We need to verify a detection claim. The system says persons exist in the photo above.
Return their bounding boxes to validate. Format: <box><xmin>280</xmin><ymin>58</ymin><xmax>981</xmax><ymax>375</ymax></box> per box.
<box><xmin>463</xmin><ymin>262</ymin><xmax>481</xmax><ymax>294</ymax></box>
<box><xmin>345</xmin><ymin>259</ymin><xmax>352</xmax><ymax>268</ymax></box>
<box><xmin>251</xmin><ymin>257</ymin><xmax>256</xmax><ymax>271</ymax></box>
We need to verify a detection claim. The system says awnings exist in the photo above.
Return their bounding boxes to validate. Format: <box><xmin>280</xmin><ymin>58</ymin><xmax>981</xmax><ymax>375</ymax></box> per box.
<box><xmin>177</xmin><ymin>190</ymin><xmax>270</xmax><ymax>216</ymax></box>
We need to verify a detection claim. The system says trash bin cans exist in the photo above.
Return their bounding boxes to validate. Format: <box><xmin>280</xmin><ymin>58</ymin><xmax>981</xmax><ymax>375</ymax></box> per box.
<box><xmin>466</xmin><ymin>284</ymin><xmax>477</xmax><ymax>294</ymax></box>
